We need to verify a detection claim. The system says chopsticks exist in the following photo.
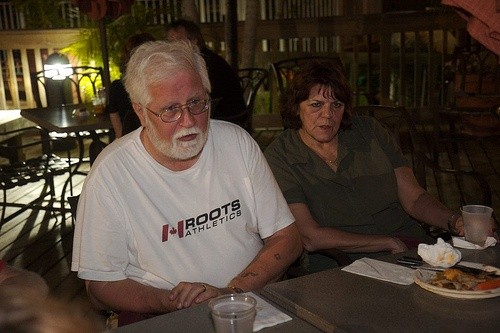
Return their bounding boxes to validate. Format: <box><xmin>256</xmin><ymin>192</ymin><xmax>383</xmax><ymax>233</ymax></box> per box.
<box><xmin>398</xmin><ymin>255</ymin><xmax>500</xmax><ymax>278</ymax></box>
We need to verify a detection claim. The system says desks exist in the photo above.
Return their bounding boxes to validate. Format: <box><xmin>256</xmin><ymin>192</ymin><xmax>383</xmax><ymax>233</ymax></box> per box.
<box><xmin>20</xmin><ymin>101</ymin><xmax>115</xmax><ymax>225</ymax></box>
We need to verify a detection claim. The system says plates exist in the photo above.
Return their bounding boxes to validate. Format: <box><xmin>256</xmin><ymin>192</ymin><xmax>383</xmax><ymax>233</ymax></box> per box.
<box><xmin>412</xmin><ymin>261</ymin><xmax>500</xmax><ymax>299</ymax></box>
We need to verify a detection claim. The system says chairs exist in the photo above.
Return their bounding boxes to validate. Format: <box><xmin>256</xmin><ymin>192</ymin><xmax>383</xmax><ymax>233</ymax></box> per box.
<box><xmin>0</xmin><ymin>51</ymin><xmax>500</xmax><ymax>327</ymax></box>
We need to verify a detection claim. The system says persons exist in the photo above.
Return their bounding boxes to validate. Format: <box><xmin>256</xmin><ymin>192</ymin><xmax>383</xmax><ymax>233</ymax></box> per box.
<box><xmin>109</xmin><ymin>32</ymin><xmax>154</xmax><ymax>139</ymax></box>
<box><xmin>165</xmin><ymin>20</ymin><xmax>252</xmax><ymax>132</ymax></box>
<box><xmin>263</xmin><ymin>59</ymin><xmax>500</xmax><ymax>277</ymax></box>
<box><xmin>70</xmin><ymin>39</ymin><xmax>303</xmax><ymax>327</ymax></box>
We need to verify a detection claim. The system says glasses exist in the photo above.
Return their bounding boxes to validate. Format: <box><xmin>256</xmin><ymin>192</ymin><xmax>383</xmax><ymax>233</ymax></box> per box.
<box><xmin>138</xmin><ymin>88</ymin><xmax>211</xmax><ymax>122</ymax></box>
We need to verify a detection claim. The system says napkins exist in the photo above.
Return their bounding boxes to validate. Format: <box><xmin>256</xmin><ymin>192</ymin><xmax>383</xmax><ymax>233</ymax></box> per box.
<box><xmin>451</xmin><ymin>236</ymin><xmax>498</xmax><ymax>250</ymax></box>
<box><xmin>417</xmin><ymin>238</ymin><xmax>462</xmax><ymax>269</ymax></box>
<box><xmin>208</xmin><ymin>292</ymin><xmax>293</xmax><ymax>332</ymax></box>
<box><xmin>341</xmin><ymin>257</ymin><xmax>418</xmax><ymax>286</ymax></box>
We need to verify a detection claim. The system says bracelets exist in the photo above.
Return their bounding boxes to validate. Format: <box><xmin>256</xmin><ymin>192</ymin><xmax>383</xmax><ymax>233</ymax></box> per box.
<box><xmin>231</xmin><ymin>287</ymin><xmax>243</xmax><ymax>293</ymax></box>
<box><xmin>448</xmin><ymin>214</ymin><xmax>457</xmax><ymax>232</ymax></box>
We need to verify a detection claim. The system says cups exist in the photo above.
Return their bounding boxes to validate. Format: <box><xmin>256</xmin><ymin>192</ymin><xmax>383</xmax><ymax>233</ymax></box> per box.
<box><xmin>90</xmin><ymin>96</ymin><xmax>104</xmax><ymax>117</ymax></box>
<box><xmin>208</xmin><ymin>294</ymin><xmax>257</xmax><ymax>333</ymax></box>
<box><xmin>460</xmin><ymin>205</ymin><xmax>493</xmax><ymax>247</ymax></box>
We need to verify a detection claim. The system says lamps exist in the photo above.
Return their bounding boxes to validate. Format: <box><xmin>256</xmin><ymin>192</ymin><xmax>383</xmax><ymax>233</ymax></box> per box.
<box><xmin>42</xmin><ymin>53</ymin><xmax>74</xmax><ymax>81</ymax></box>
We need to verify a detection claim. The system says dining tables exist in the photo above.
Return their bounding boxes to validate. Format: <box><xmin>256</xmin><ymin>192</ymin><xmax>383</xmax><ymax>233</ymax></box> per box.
<box><xmin>106</xmin><ymin>231</ymin><xmax>500</xmax><ymax>333</ymax></box>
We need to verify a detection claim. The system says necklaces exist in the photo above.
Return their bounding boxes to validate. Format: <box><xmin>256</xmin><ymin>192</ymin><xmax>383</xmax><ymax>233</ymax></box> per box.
<box><xmin>329</xmin><ymin>159</ymin><xmax>336</xmax><ymax>168</ymax></box>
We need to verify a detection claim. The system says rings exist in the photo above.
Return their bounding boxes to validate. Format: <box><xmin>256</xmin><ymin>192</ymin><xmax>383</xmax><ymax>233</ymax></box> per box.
<box><xmin>203</xmin><ymin>285</ymin><xmax>206</xmax><ymax>291</ymax></box>
